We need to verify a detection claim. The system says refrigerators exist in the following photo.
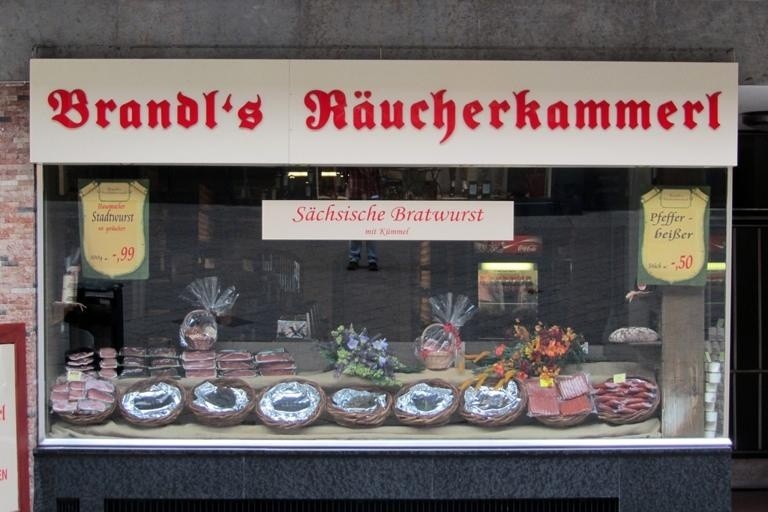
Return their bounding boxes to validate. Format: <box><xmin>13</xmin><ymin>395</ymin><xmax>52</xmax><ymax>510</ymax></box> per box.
<box><xmin>467</xmin><ymin>231</ymin><xmax>544</xmax><ymax>344</ymax></box>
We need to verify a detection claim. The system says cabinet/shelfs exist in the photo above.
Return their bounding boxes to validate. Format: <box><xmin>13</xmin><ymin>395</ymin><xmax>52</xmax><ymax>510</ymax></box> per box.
<box><xmin>30</xmin><ymin>165</ymin><xmax>734</xmax><ymax>511</ymax></box>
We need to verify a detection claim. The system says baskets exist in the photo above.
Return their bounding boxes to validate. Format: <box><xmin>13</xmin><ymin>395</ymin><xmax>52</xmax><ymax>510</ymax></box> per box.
<box><xmin>458</xmin><ymin>376</ymin><xmax>529</xmax><ymax>426</ymax></box>
<box><xmin>326</xmin><ymin>386</ymin><xmax>393</xmax><ymax>428</ymax></box>
<box><xmin>255</xmin><ymin>378</ymin><xmax>326</xmax><ymax>429</ymax></box>
<box><xmin>117</xmin><ymin>377</ymin><xmax>187</xmax><ymax>427</ymax></box>
<box><xmin>592</xmin><ymin>376</ymin><xmax>661</xmax><ymax>425</ymax></box>
<box><xmin>391</xmin><ymin>377</ymin><xmax>459</xmax><ymax>426</ymax></box>
<box><xmin>180</xmin><ymin>310</ymin><xmax>218</xmax><ymax>351</ymax></box>
<box><xmin>527</xmin><ymin>376</ymin><xmax>599</xmax><ymax>429</ymax></box>
<box><xmin>184</xmin><ymin>377</ymin><xmax>256</xmax><ymax>428</ymax></box>
<box><xmin>419</xmin><ymin>323</ymin><xmax>459</xmax><ymax>370</ymax></box>
<box><xmin>58</xmin><ymin>377</ymin><xmax>121</xmax><ymax>426</ymax></box>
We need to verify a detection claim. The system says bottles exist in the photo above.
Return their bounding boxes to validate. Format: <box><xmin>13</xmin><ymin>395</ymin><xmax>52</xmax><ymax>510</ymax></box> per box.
<box><xmin>489</xmin><ymin>273</ymin><xmax>534</xmax><ymax>303</ymax></box>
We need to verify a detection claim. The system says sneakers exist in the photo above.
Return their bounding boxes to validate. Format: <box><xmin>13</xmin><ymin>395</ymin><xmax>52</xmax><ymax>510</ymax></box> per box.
<box><xmin>368</xmin><ymin>262</ymin><xmax>379</xmax><ymax>272</ymax></box>
<box><xmin>346</xmin><ymin>260</ymin><xmax>359</xmax><ymax>271</ymax></box>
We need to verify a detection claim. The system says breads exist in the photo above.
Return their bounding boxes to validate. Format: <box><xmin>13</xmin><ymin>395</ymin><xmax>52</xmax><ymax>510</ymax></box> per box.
<box><xmin>608</xmin><ymin>327</ymin><xmax>658</xmax><ymax>343</ymax></box>
